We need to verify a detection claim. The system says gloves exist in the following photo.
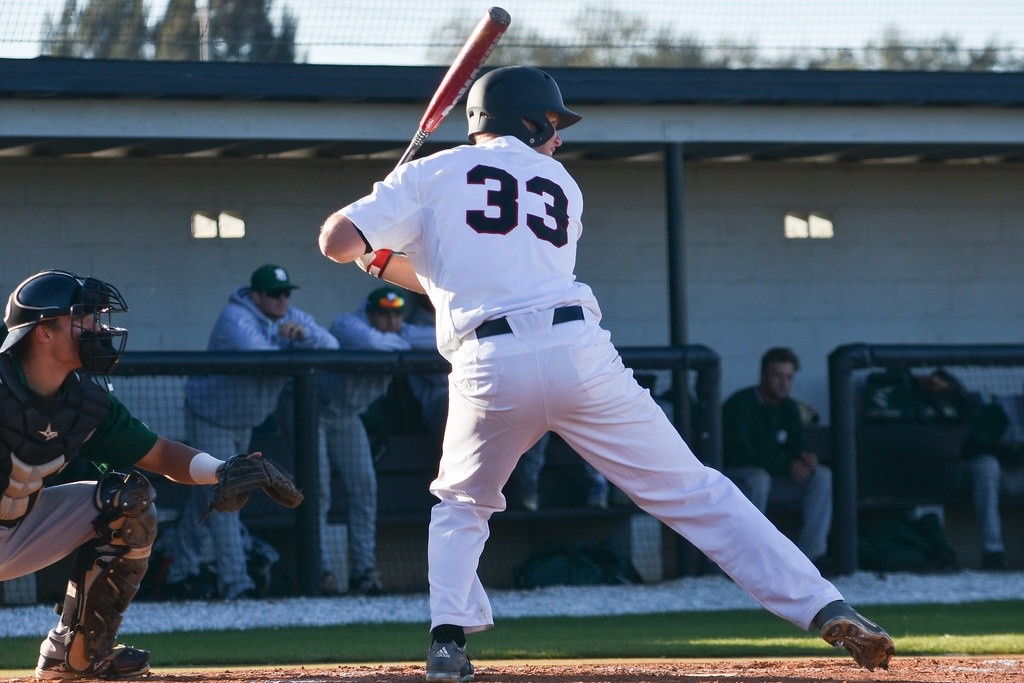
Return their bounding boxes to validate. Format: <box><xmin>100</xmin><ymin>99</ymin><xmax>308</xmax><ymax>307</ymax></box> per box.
<box><xmin>355</xmin><ymin>249</ymin><xmax>393</xmax><ymax>278</ymax></box>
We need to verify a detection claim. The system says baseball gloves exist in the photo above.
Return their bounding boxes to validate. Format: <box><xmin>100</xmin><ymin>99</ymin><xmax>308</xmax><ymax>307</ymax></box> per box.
<box><xmin>195</xmin><ymin>451</ymin><xmax>305</xmax><ymax>529</ymax></box>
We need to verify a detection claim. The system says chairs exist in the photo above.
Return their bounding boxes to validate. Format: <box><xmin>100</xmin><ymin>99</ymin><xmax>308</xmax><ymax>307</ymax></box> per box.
<box><xmin>370</xmin><ymin>394</ymin><xmax>1024</xmax><ymax>524</ymax></box>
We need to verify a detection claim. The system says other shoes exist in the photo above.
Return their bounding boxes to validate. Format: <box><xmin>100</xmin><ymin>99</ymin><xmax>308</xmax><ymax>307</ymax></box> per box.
<box><xmin>588</xmin><ymin>482</ymin><xmax>610</xmax><ymax>509</ymax></box>
<box><xmin>516</xmin><ymin>477</ymin><xmax>538</xmax><ymax>509</ymax></box>
<box><xmin>322</xmin><ymin>573</ymin><xmax>339</xmax><ymax>596</ymax></box>
<box><xmin>349</xmin><ymin>568</ymin><xmax>395</xmax><ymax>596</ymax></box>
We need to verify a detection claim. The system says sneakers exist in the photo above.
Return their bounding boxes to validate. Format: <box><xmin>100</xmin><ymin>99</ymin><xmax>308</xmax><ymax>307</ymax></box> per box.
<box><xmin>35</xmin><ymin>654</ymin><xmax>150</xmax><ymax>680</ymax></box>
<box><xmin>813</xmin><ymin>600</ymin><xmax>896</xmax><ymax>672</ymax></box>
<box><xmin>426</xmin><ymin>640</ymin><xmax>475</xmax><ymax>683</ymax></box>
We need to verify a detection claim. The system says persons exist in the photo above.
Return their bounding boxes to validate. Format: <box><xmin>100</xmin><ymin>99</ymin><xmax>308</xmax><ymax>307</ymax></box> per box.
<box><xmin>320</xmin><ymin>67</ymin><xmax>896</xmax><ymax>683</ymax></box>
<box><xmin>722</xmin><ymin>347</ymin><xmax>832</xmax><ymax>569</ymax></box>
<box><xmin>855</xmin><ymin>367</ymin><xmax>1024</xmax><ymax>572</ymax></box>
<box><xmin>401</xmin><ymin>291</ymin><xmax>608</xmax><ymax>512</ymax></box>
<box><xmin>0</xmin><ymin>270</ymin><xmax>263</xmax><ymax>678</ymax></box>
<box><xmin>161</xmin><ymin>264</ymin><xmax>340</xmax><ymax>603</ymax></box>
<box><xmin>279</xmin><ymin>287</ymin><xmax>412</xmax><ymax>597</ymax></box>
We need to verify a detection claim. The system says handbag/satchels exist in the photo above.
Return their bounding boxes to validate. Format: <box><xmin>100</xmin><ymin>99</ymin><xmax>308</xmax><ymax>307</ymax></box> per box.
<box><xmin>512</xmin><ymin>539</ymin><xmax>643</xmax><ymax>588</ymax></box>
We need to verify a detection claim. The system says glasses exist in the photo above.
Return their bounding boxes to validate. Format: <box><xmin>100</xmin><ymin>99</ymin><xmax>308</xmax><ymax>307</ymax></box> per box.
<box><xmin>265</xmin><ymin>288</ymin><xmax>289</xmax><ymax>299</ymax></box>
<box><xmin>376</xmin><ymin>296</ymin><xmax>406</xmax><ymax>309</ymax></box>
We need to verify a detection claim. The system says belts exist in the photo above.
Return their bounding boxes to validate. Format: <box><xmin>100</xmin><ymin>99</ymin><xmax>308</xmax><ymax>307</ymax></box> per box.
<box><xmin>475</xmin><ymin>306</ymin><xmax>584</xmax><ymax>339</ymax></box>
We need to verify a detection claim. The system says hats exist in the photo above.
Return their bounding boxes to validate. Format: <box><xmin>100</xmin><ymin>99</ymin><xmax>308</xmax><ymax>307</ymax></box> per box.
<box><xmin>251</xmin><ymin>263</ymin><xmax>298</xmax><ymax>293</ymax></box>
<box><xmin>366</xmin><ymin>287</ymin><xmax>407</xmax><ymax>313</ymax></box>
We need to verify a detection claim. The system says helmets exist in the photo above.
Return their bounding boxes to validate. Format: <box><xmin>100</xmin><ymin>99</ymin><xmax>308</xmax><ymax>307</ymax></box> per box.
<box><xmin>466</xmin><ymin>65</ymin><xmax>582</xmax><ymax>145</ymax></box>
<box><xmin>0</xmin><ymin>268</ymin><xmax>84</xmax><ymax>354</ymax></box>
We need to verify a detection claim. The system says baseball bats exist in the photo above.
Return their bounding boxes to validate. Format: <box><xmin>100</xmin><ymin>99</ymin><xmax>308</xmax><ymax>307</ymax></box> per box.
<box><xmin>396</xmin><ymin>5</ymin><xmax>512</xmax><ymax>170</ymax></box>
